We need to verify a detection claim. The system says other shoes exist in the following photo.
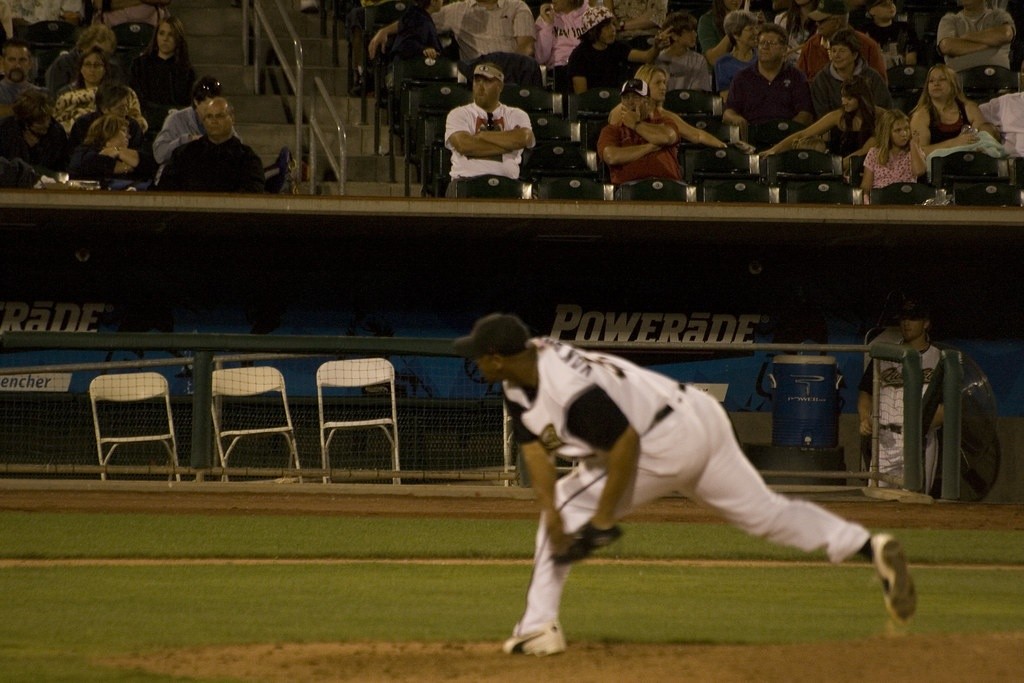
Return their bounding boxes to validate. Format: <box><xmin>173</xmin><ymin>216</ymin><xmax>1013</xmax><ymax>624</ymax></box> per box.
<box><xmin>266</xmin><ymin>146</ymin><xmax>290</xmax><ymax>195</ymax></box>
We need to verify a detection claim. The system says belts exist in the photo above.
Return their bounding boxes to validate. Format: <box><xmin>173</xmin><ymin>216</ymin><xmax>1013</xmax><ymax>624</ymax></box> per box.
<box><xmin>880</xmin><ymin>423</ymin><xmax>902</xmax><ymax>434</ymax></box>
<box><xmin>653</xmin><ymin>382</ymin><xmax>686</xmax><ymax>426</ymax></box>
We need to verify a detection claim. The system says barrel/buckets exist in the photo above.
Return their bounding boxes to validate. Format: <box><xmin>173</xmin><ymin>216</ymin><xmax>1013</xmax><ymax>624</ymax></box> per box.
<box><xmin>771</xmin><ymin>355</ymin><xmax>841</xmax><ymax>448</ymax></box>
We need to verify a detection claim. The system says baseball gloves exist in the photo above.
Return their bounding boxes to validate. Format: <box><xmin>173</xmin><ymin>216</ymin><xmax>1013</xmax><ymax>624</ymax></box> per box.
<box><xmin>549</xmin><ymin>517</ymin><xmax>625</xmax><ymax>564</ymax></box>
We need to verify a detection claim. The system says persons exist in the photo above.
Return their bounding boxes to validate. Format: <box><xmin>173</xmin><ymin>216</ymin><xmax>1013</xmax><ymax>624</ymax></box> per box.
<box><xmin>861</xmin><ymin>109</ymin><xmax>931</xmax><ymax>206</ymax></box>
<box><xmin>453</xmin><ymin>314</ymin><xmax>918</xmax><ymax>658</ymax></box>
<box><xmin>298</xmin><ymin>0</ymin><xmax>1024</xmax><ymax>171</ymax></box>
<box><xmin>859</xmin><ymin>299</ymin><xmax>946</xmax><ymax>494</ymax></box>
<box><xmin>597</xmin><ymin>78</ymin><xmax>683</xmax><ymax>203</ymax></box>
<box><xmin>0</xmin><ymin>0</ymin><xmax>268</xmax><ymax>195</ymax></box>
<box><xmin>444</xmin><ymin>61</ymin><xmax>536</xmax><ymax>200</ymax></box>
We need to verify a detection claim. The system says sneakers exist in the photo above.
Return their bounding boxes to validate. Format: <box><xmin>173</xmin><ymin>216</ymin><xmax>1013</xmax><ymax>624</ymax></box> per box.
<box><xmin>502</xmin><ymin>624</ymin><xmax>567</xmax><ymax>656</ymax></box>
<box><xmin>869</xmin><ymin>533</ymin><xmax>918</xmax><ymax>623</ymax></box>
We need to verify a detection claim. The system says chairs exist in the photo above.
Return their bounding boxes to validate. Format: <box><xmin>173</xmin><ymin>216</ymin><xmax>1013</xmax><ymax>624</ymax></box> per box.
<box><xmin>316</xmin><ymin>358</ymin><xmax>402</xmax><ymax>486</ymax></box>
<box><xmin>88</xmin><ymin>371</ymin><xmax>182</xmax><ymax>483</ymax></box>
<box><xmin>319</xmin><ymin>0</ymin><xmax>1024</xmax><ymax>208</ymax></box>
<box><xmin>209</xmin><ymin>366</ymin><xmax>303</xmax><ymax>483</ymax></box>
<box><xmin>23</xmin><ymin>20</ymin><xmax>156</xmax><ymax>87</ymax></box>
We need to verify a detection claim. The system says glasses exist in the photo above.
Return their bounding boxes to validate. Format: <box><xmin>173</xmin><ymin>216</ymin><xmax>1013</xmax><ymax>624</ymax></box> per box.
<box><xmin>82</xmin><ymin>61</ymin><xmax>104</xmax><ymax>70</ymax></box>
<box><xmin>200</xmin><ymin>80</ymin><xmax>222</xmax><ymax>93</ymax></box>
<box><xmin>486</xmin><ymin>113</ymin><xmax>495</xmax><ymax>130</ymax></box>
<box><xmin>755</xmin><ymin>39</ymin><xmax>782</xmax><ymax>49</ymax></box>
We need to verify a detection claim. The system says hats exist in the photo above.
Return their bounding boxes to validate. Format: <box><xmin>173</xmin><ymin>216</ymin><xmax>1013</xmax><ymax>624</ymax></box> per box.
<box><xmin>473</xmin><ymin>64</ymin><xmax>505</xmax><ymax>83</ymax></box>
<box><xmin>808</xmin><ymin>0</ymin><xmax>849</xmax><ymax>21</ymax></box>
<box><xmin>577</xmin><ymin>6</ymin><xmax>613</xmax><ymax>39</ymax></box>
<box><xmin>619</xmin><ymin>79</ymin><xmax>651</xmax><ymax>97</ymax></box>
<box><xmin>893</xmin><ymin>296</ymin><xmax>929</xmax><ymax>321</ymax></box>
<box><xmin>453</xmin><ymin>313</ymin><xmax>530</xmax><ymax>356</ymax></box>
